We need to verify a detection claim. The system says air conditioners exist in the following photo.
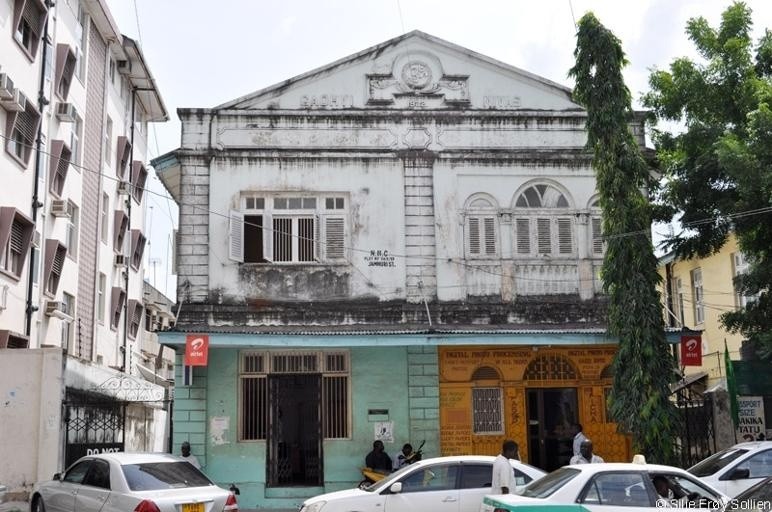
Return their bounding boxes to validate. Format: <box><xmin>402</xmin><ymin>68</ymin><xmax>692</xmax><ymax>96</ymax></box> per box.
<box><xmin>0</xmin><ymin>86</ymin><xmax>28</xmax><ymax>114</ymax></box>
<box><xmin>44</xmin><ymin>300</ymin><xmax>67</xmax><ymax>317</ymax></box>
<box><xmin>114</xmin><ymin>254</ymin><xmax>129</xmax><ymax>268</ymax></box>
<box><xmin>50</xmin><ymin>197</ymin><xmax>74</xmax><ymax>218</ymax></box>
<box><xmin>0</xmin><ymin>72</ymin><xmax>15</xmax><ymax>100</ymax></box>
<box><xmin>117</xmin><ymin>179</ymin><xmax>131</xmax><ymax>197</ymax></box>
<box><xmin>55</xmin><ymin>102</ymin><xmax>78</xmax><ymax>123</ymax></box>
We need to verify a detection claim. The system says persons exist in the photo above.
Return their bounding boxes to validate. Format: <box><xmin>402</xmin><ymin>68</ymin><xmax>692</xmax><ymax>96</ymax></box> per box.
<box><xmin>492</xmin><ymin>440</ymin><xmax>517</xmax><ymax>512</ymax></box>
<box><xmin>178</xmin><ymin>442</ymin><xmax>201</xmax><ymax>473</ymax></box>
<box><xmin>571</xmin><ymin>423</ymin><xmax>588</xmax><ymax>455</ymax></box>
<box><xmin>365</xmin><ymin>440</ymin><xmax>392</xmax><ymax>485</ymax></box>
<box><xmin>393</xmin><ymin>444</ymin><xmax>421</xmax><ymax>472</ymax></box>
<box><xmin>569</xmin><ymin>439</ymin><xmax>605</xmax><ymax>465</ymax></box>
<box><xmin>652</xmin><ymin>474</ymin><xmax>699</xmax><ymax>508</ymax></box>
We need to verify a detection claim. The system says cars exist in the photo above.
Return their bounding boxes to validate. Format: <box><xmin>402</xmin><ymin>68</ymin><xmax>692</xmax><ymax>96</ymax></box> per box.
<box><xmin>481</xmin><ymin>453</ymin><xmax>735</xmax><ymax>512</ymax></box>
<box><xmin>713</xmin><ymin>475</ymin><xmax>772</xmax><ymax>512</ymax></box>
<box><xmin>27</xmin><ymin>451</ymin><xmax>238</xmax><ymax>512</ymax></box>
<box><xmin>623</xmin><ymin>440</ymin><xmax>772</xmax><ymax>502</ymax></box>
<box><xmin>299</xmin><ymin>454</ymin><xmax>551</xmax><ymax>512</ymax></box>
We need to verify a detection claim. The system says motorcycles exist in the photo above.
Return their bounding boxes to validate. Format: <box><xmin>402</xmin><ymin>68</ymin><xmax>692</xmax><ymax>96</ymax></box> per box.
<box><xmin>360</xmin><ymin>439</ymin><xmax>434</xmax><ymax>492</ymax></box>
